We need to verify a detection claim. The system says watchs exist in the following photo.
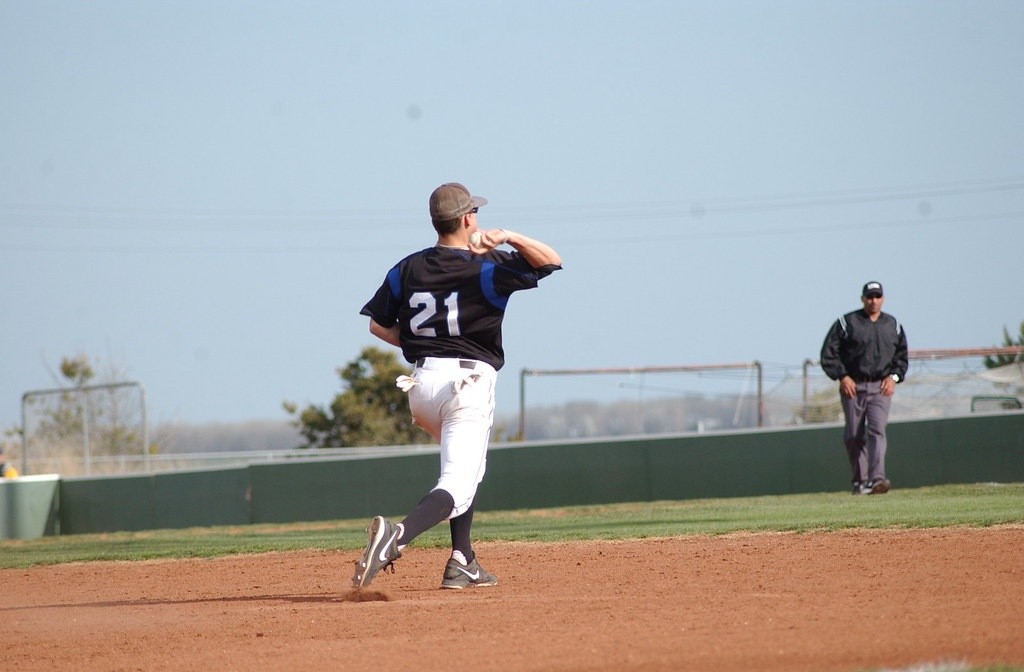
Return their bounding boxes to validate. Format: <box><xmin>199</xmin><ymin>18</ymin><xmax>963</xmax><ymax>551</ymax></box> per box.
<box><xmin>889</xmin><ymin>374</ymin><xmax>900</xmax><ymax>383</ymax></box>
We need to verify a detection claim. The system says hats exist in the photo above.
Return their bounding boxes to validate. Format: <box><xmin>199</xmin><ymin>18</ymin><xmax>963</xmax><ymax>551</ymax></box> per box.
<box><xmin>429</xmin><ymin>182</ymin><xmax>489</xmax><ymax>223</ymax></box>
<box><xmin>863</xmin><ymin>281</ymin><xmax>883</xmax><ymax>298</ymax></box>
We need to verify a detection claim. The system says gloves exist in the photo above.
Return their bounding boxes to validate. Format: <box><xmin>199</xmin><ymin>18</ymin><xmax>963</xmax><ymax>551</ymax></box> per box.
<box><xmin>394</xmin><ymin>373</ymin><xmax>417</xmax><ymax>391</ymax></box>
<box><xmin>453</xmin><ymin>378</ymin><xmax>474</xmax><ymax>394</ymax></box>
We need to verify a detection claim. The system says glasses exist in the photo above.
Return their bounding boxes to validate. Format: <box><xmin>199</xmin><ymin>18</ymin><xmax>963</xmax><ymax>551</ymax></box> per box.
<box><xmin>470</xmin><ymin>207</ymin><xmax>478</xmax><ymax>214</ymax></box>
<box><xmin>864</xmin><ymin>293</ymin><xmax>881</xmax><ymax>298</ymax></box>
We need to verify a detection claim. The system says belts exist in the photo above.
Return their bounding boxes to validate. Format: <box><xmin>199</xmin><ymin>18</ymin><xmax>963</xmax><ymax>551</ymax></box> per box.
<box><xmin>414</xmin><ymin>359</ymin><xmax>475</xmax><ymax>369</ymax></box>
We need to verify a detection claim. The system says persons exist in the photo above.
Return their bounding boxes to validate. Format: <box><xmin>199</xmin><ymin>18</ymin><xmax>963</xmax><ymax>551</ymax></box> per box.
<box><xmin>819</xmin><ymin>281</ymin><xmax>909</xmax><ymax>493</ymax></box>
<box><xmin>353</xmin><ymin>183</ymin><xmax>565</xmax><ymax>592</ymax></box>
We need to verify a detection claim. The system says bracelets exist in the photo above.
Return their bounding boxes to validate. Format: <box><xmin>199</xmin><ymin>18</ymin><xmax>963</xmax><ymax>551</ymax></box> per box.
<box><xmin>499</xmin><ymin>228</ymin><xmax>507</xmax><ymax>246</ymax></box>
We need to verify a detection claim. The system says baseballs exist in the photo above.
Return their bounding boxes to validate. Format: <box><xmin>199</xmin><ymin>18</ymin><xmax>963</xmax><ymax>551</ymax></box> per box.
<box><xmin>469</xmin><ymin>231</ymin><xmax>483</xmax><ymax>248</ymax></box>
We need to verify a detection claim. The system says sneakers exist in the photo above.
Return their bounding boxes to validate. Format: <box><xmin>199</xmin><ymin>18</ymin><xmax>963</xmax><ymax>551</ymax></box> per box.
<box><xmin>852</xmin><ymin>480</ymin><xmax>866</xmax><ymax>494</ymax></box>
<box><xmin>441</xmin><ymin>551</ymin><xmax>497</xmax><ymax>589</ymax></box>
<box><xmin>864</xmin><ymin>479</ymin><xmax>889</xmax><ymax>494</ymax></box>
<box><xmin>351</xmin><ymin>516</ymin><xmax>402</xmax><ymax>590</ymax></box>
